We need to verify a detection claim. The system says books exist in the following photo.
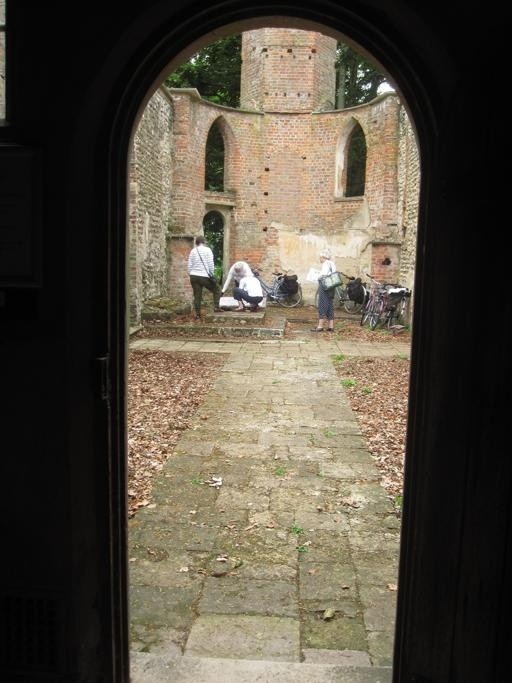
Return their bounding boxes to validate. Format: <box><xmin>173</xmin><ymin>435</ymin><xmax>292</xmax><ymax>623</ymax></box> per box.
<box><xmin>306</xmin><ymin>267</ymin><xmax>320</xmax><ymax>283</ymax></box>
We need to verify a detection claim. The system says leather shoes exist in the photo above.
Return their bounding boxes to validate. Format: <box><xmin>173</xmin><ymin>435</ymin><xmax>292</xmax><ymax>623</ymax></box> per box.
<box><xmin>310</xmin><ymin>327</ymin><xmax>334</xmax><ymax>332</ymax></box>
<box><xmin>214</xmin><ymin>307</ymin><xmax>257</xmax><ymax>313</ymax></box>
<box><xmin>197</xmin><ymin>315</ymin><xmax>202</xmax><ymax>318</ymax></box>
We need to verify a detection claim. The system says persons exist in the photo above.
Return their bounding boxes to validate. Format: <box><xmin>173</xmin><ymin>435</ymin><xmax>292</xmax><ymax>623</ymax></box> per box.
<box><xmin>311</xmin><ymin>250</ymin><xmax>337</xmax><ymax>331</ymax></box>
<box><xmin>187</xmin><ymin>235</ymin><xmax>224</xmax><ymax>319</ymax></box>
<box><xmin>222</xmin><ymin>260</ymin><xmax>254</xmax><ymax>295</ymax></box>
<box><xmin>232</xmin><ymin>270</ymin><xmax>263</xmax><ymax>310</ymax></box>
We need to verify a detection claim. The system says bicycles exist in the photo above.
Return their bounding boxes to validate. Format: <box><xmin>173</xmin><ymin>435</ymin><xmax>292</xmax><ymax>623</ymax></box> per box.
<box><xmin>314</xmin><ymin>271</ymin><xmax>412</xmax><ymax>330</ymax></box>
<box><xmin>251</xmin><ymin>266</ymin><xmax>302</xmax><ymax>307</ymax></box>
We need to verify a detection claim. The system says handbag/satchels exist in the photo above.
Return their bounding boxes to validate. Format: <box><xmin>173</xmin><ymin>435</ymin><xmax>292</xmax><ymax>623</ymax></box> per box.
<box><xmin>210</xmin><ymin>277</ymin><xmax>217</xmax><ymax>283</ymax></box>
<box><xmin>320</xmin><ymin>272</ymin><xmax>343</xmax><ymax>292</ymax></box>
<box><xmin>347</xmin><ymin>278</ymin><xmax>368</xmax><ymax>305</ymax></box>
<box><xmin>279</xmin><ymin>275</ymin><xmax>298</xmax><ymax>294</ymax></box>
<box><xmin>384</xmin><ymin>290</ymin><xmax>405</xmax><ymax>312</ymax></box>
